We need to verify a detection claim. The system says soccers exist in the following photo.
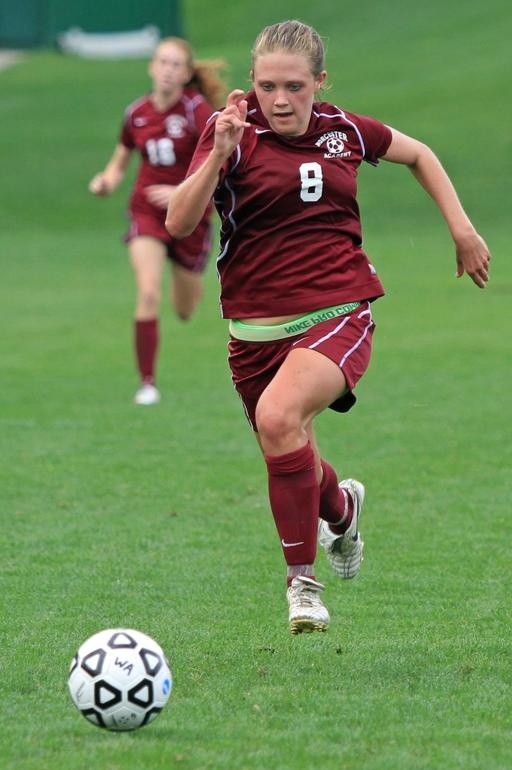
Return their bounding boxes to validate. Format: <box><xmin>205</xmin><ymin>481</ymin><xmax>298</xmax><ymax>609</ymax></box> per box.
<box><xmin>68</xmin><ymin>628</ymin><xmax>172</xmax><ymax>731</ymax></box>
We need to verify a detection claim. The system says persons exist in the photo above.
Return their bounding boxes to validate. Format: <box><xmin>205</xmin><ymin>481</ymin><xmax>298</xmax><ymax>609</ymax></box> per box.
<box><xmin>90</xmin><ymin>35</ymin><xmax>228</xmax><ymax>405</ymax></box>
<box><xmin>165</xmin><ymin>19</ymin><xmax>490</xmax><ymax>635</ymax></box>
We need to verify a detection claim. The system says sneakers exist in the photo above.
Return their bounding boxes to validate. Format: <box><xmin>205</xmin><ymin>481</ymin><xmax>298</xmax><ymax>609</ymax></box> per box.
<box><xmin>135</xmin><ymin>384</ymin><xmax>160</xmax><ymax>405</ymax></box>
<box><xmin>318</xmin><ymin>478</ymin><xmax>364</xmax><ymax>580</ymax></box>
<box><xmin>286</xmin><ymin>575</ymin><xmax>330</xmax><ymax>636</ymax></box>
